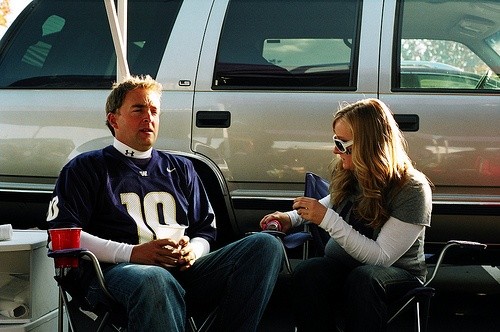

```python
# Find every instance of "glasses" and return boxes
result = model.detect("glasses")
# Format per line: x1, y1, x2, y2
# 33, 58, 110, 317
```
332, 134, 353, 153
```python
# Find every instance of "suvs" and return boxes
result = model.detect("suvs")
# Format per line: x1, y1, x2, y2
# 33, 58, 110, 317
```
1, 0, 500, 255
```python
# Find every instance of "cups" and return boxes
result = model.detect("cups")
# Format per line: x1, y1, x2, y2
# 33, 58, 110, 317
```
155, 225, 188, 267
49, 228, 83, 268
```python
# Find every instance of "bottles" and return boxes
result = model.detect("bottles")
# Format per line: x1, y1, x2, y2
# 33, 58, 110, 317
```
262, 220, 281, 231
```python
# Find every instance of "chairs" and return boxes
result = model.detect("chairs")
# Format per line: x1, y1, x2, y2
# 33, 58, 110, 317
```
46, 248, 223, 332
261, 171, 489, 332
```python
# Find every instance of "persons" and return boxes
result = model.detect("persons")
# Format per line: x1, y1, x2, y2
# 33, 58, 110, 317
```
259, 97, 432, 332
46, 74, 284, 332
